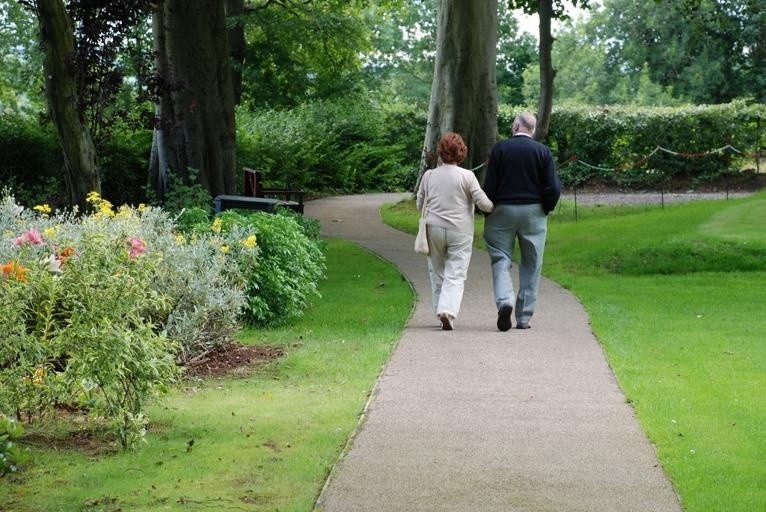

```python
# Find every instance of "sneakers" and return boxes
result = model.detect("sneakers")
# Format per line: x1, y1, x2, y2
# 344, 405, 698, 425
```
516, 321, 531, 329
439, 312, 454, 330
497, 303, 513, 331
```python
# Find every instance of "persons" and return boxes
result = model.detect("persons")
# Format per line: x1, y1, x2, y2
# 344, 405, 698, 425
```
415, 132, 494, 330
483, 111, 560, 331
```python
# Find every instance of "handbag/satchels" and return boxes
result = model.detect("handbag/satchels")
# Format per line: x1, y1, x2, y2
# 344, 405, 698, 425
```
414, 218, 429, 255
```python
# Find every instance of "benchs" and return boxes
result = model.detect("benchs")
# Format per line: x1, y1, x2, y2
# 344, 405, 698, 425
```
243, 167, 305, 215
